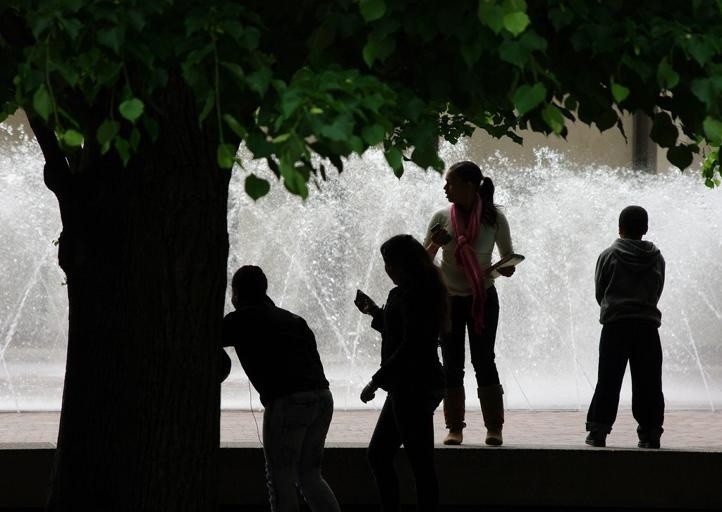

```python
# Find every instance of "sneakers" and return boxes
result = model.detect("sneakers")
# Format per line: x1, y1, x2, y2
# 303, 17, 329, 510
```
637, 437, 662, 451
584, 429, 608, 449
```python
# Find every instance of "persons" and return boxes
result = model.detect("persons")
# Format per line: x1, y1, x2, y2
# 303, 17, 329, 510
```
221, 263, 343, 512
420, 160, 516, 446
354, 235, 453, 510
585, 205, 666, 450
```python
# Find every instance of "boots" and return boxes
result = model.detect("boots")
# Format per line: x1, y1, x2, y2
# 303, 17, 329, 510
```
443, 385, 467, 445
478, 383, 505, 446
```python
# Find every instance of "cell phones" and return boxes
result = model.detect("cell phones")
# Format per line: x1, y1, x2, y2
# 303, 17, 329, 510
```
430, 222, 453, 246
